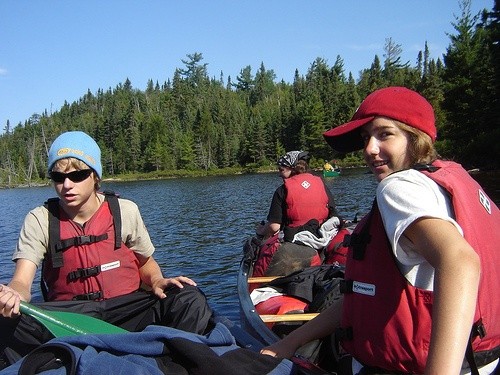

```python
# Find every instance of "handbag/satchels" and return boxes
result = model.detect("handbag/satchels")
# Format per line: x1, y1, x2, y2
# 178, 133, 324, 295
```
253, 228, 354, 315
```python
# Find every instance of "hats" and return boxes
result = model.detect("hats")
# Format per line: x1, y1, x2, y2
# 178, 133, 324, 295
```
322, 86, 437, 153
47, 131, 102, 179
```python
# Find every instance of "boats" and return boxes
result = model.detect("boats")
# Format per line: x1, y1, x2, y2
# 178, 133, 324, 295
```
236, 237, 342, 375
0, 300, 311, 375
322, 169, 341, 177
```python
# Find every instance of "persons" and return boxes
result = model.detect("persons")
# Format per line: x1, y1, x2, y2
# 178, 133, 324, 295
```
322, 160, 340, 172
260, 86, 500, 375
255, 151, 338, 243
0, 131, 214, 365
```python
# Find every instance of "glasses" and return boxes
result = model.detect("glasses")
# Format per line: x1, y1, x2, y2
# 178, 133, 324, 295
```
49, 168, 94, 183
